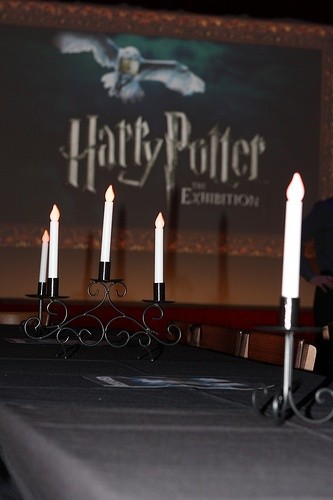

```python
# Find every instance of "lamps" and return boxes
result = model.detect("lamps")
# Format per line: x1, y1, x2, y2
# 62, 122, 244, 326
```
21, 183, 183, 349
250, 172, 332, 425
